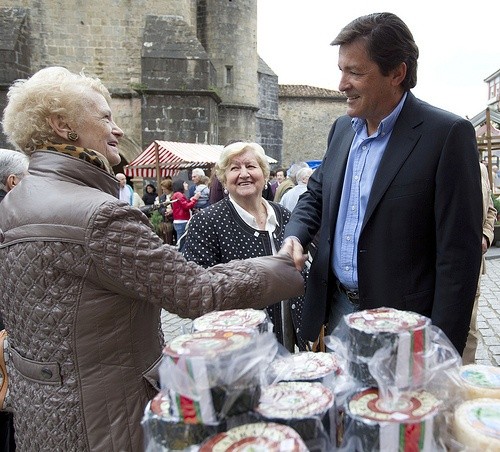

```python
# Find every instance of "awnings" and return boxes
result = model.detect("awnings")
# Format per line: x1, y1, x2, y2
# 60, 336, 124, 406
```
123, 140, 280, 202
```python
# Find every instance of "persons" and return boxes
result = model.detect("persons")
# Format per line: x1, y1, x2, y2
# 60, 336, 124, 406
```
463, 161, 498, 364
276, 11, 484, 361
179, 141, 309, 353
0, 148, 29, 203
115, 162, 319, 248
0, 67, 308, 452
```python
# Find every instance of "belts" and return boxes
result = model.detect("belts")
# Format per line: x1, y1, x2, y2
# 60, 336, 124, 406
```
335, 278, 359, 305
